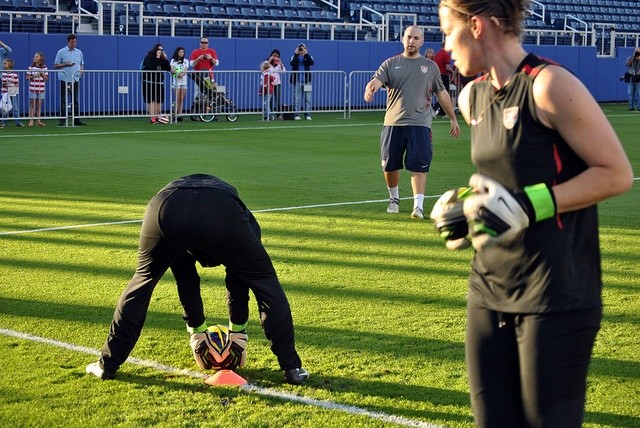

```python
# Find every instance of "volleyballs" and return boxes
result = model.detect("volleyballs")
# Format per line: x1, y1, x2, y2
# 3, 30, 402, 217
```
204, 324, 240, 372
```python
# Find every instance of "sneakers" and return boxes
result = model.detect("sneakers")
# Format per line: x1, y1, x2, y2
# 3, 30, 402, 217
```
411, 208, 424, 219
28, 123, 34, 126
75, 121, 86, 126
16, 124, 25, 127
150, 118, 157, 124
295, 116, 301, 120
306, 116, 312, 120
58, 123, 65, 126
86, 361, 115, 379
0, 124, 6, 128
37, 123, 45, 126
172, 117, 183, 121
284, 368, 309, 385
387, 198, 399, 213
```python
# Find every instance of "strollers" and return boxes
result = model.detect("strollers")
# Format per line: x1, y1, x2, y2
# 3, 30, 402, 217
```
188, 69, 238, 122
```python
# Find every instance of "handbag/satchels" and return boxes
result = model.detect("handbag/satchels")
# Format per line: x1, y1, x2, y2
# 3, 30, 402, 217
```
283, 113, 294, 120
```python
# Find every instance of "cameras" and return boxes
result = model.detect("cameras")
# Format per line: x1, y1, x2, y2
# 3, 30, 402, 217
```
635, 51, 640, 58
298, 47, 303, 51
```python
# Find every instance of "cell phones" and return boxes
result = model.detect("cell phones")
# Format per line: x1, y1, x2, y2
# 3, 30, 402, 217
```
71, 63, 75, 65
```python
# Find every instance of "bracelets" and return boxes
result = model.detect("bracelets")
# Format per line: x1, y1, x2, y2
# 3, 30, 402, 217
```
1, 44, 5, 47
452, 71, 455, 74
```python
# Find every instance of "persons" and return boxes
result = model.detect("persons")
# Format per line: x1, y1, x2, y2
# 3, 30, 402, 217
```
87, 173, 310, 384
625, 48, 640, 111
190, 37, 219, 121
259, 49, 286, 121
434, 42, 455, 118
26, 52, 48, 127
54, 35, 87, 126
364, 25, 460, 220
290, 43, 314, 121
2, 59, 25, 128
429, 0, 634, 428
259, 63, 276, 120
169, 47, 189, 121
423, 48, 439, 119
0, 39, 12, 53
142, 44, 171, 124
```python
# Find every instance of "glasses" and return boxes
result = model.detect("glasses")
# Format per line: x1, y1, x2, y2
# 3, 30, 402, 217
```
157, 49, 163, 51
201, 42, 208, 44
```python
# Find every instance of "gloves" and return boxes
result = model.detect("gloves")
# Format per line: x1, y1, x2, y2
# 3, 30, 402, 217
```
221, 321, 248, 369
430, 187, 471, 251
463, 174, 556, 252
187, 320, 220, 370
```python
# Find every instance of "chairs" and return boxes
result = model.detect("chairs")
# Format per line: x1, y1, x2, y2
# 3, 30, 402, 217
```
1, 0, 80, 34
347, 0, 589, 45
85, 1, 372, 41
